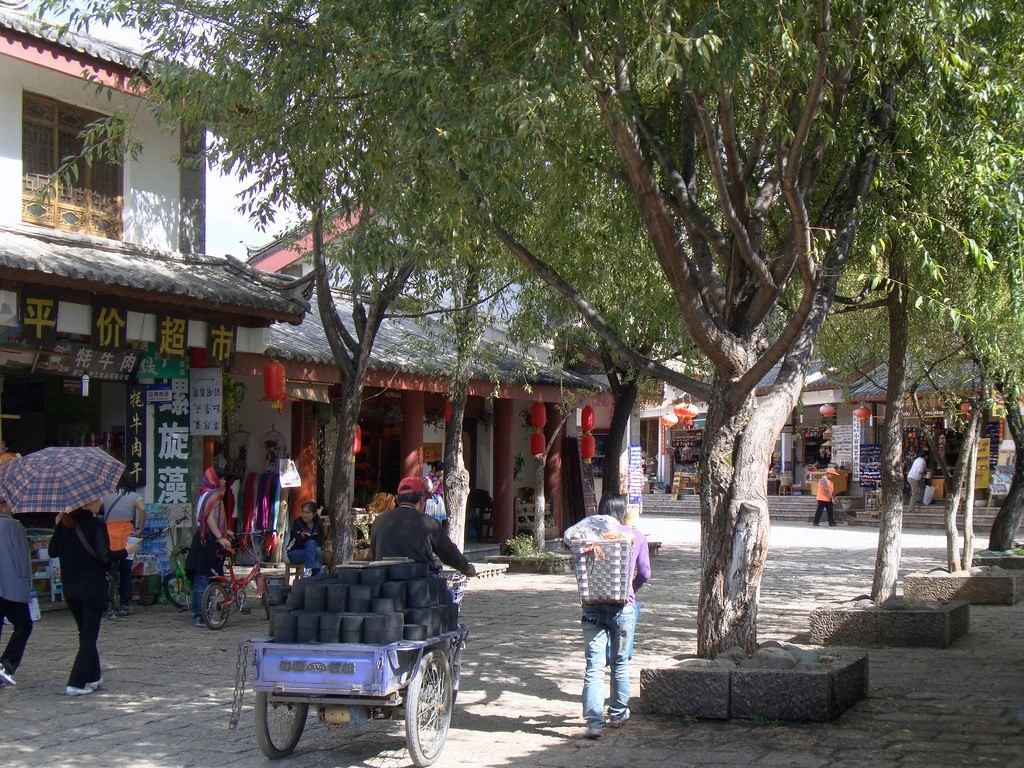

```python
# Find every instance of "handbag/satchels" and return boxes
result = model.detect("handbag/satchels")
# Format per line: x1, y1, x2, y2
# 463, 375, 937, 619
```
923, 486, 935, 505
106, 574, 114, 603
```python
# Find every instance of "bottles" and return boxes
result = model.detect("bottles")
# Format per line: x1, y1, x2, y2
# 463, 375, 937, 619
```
138, 517, 170, 573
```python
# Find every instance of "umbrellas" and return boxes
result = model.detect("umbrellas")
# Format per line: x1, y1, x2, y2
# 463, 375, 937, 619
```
1, 446, 127, 513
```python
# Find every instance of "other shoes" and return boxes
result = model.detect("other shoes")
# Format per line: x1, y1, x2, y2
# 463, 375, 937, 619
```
609, 709, 632, 728
302, 567, 314, 578
813, 522, 820, 527
118, 606, 134, 616
829, 522, 837, 526
65, 685, 94, 697
194, 618, 219, 628
0, 661, 17, 686
586, 726, 603, 737
86, 678, 104, 690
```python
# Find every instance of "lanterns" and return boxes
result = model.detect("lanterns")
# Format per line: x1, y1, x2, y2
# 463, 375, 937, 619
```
262, 359, 287, 403
529, 401, 548, 459
350, 424, 362, 459
581, 405, 597, 461
660, 401, 699, 428
444, 397, 453, 423
961, 399, 981, 419
852, 405, 873, 420
819, 403, 835, 416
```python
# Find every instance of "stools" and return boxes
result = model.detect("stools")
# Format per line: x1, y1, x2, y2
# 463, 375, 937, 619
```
284, 563, 306, 586
778, 485, 791, 496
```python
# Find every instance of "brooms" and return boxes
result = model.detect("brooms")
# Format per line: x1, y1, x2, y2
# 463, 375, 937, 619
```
832, 497, 855, 525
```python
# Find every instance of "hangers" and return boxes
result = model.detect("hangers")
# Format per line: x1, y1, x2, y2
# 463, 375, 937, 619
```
264, 424, 280, 435
232, 424, 249, 435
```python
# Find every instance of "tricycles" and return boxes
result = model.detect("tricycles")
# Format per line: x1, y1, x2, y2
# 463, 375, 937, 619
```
249, 569, 468, 768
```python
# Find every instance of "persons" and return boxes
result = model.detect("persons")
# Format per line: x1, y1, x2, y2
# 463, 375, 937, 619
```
581, 492, 652, 736
422, 460, 450, 526
812, 467, 839, 527
99, 470, 147, 617
288, 501, 328, 578
0, 495, 34, 686
184, 466, 235, 627
370, 477, 477, 579
47, 495, 143, 695
906, 450, 930, 513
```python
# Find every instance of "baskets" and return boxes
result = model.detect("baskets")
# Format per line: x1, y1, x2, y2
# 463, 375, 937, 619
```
436, 570, 465, 604
569, 539, 634, 604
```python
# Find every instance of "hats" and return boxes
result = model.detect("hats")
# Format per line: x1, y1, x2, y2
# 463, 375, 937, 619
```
398, 479, 427, 495
827, 467, 840, 477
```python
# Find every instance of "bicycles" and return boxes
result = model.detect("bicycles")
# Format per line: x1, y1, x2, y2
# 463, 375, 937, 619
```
199, 529, 277, 630
146, 515, 194, 610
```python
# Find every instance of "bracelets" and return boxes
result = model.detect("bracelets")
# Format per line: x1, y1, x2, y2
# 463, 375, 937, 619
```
217, 535, 224, 540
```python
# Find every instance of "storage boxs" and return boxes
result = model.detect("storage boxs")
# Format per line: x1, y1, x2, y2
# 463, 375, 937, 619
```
131, 573, 161, 596
136, 501, 192, 527
